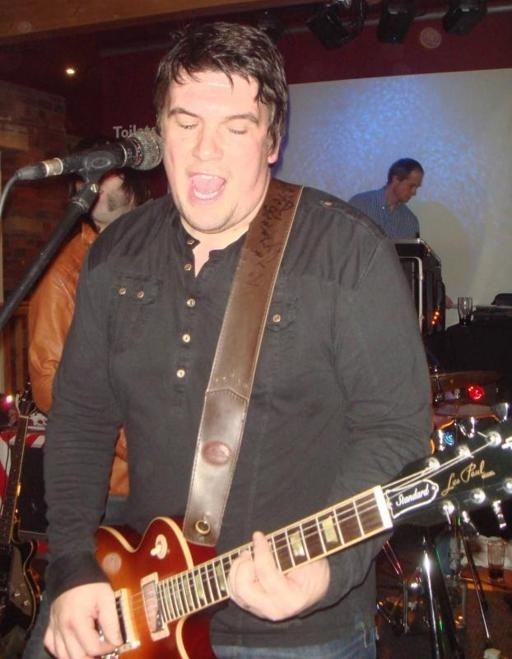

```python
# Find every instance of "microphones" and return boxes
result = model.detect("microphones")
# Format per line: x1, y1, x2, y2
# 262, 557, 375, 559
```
18, 127, 163, 182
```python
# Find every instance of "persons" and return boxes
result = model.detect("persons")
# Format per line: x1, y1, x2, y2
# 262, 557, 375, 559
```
345, 158, 425, 242
28, 131, 153, 525
43, 23, 431, 659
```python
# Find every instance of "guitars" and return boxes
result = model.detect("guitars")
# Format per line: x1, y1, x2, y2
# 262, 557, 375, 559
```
0, 380, 39, 636
94, 403, 511, 659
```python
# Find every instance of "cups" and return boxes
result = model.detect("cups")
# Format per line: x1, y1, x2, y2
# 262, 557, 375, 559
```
487, 539, 506, 583
457, 296, 473, 327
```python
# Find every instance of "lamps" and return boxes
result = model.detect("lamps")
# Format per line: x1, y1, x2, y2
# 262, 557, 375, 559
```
303, 1, 487, 50
